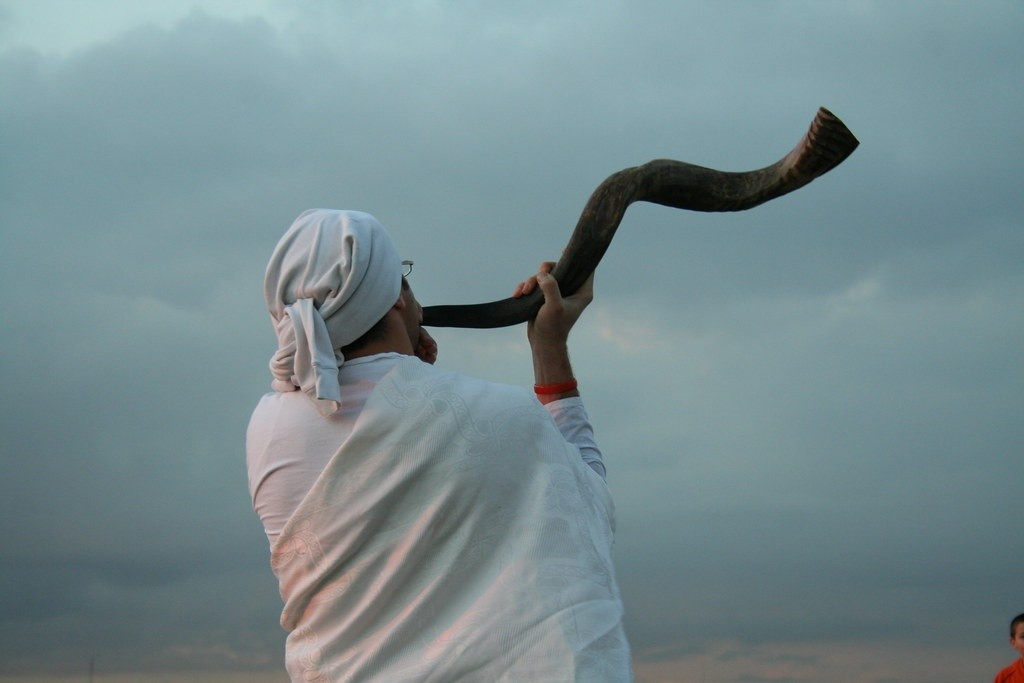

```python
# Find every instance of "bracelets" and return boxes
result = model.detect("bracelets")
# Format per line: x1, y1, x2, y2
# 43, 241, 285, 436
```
533, 377, 578, 395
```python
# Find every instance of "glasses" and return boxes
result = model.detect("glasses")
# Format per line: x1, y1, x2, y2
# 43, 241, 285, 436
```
401, 258, 414, 277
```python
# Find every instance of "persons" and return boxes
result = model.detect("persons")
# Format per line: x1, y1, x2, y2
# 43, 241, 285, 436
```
994, 613, 1024, 683
244, 209, 631, 683
